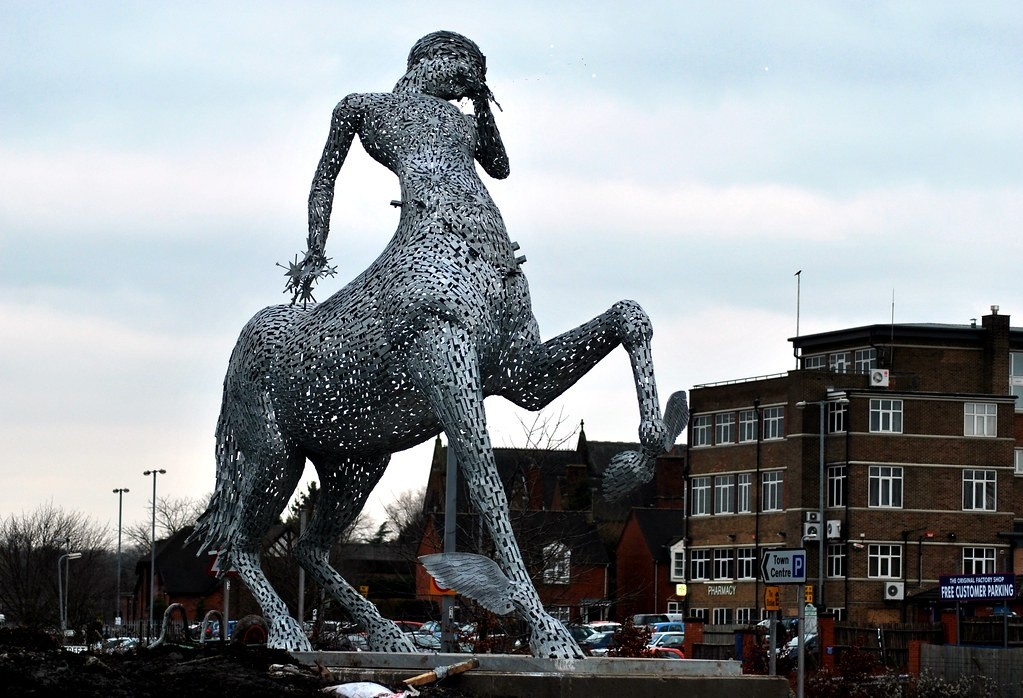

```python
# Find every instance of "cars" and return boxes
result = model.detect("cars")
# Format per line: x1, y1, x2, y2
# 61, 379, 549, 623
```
56, 630, 156, 657
187, 609, 685, 662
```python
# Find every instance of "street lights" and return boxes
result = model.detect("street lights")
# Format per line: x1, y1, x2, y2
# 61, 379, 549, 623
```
793, 398, 852, 607
111, 488, 130, 626
57, 553, 82, 628
55, 538, 68, 632
143, 469, 165, 636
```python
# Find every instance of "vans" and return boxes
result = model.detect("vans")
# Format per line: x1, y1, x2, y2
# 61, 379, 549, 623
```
765, 633, 820, 673
756, 614, 798, 644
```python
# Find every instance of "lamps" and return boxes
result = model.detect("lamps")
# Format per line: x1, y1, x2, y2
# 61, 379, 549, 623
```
948, 533, 956, 539
777, 532, 786, 537
727, 535, 736, 541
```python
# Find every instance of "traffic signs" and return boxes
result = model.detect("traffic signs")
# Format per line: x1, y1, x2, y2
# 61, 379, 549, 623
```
759, 547, 806, 584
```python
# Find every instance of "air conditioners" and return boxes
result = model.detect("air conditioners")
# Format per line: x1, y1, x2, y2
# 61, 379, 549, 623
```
803, 523, 820, 540
869, 369, 889, 387
884, 582, 904, 600
826, 520, 841, 538
806, 512, 820, 522
